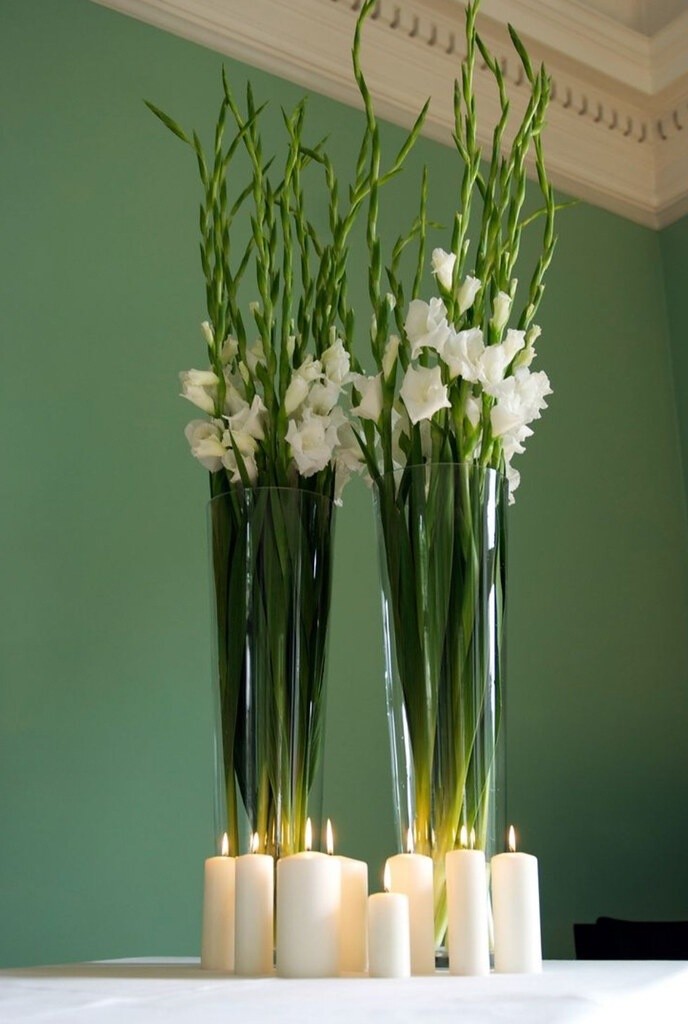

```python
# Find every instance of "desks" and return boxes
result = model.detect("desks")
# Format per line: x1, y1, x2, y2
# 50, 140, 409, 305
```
0, 956, 688, 1024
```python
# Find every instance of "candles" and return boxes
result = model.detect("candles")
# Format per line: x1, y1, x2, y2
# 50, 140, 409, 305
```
200, 816, 543, 978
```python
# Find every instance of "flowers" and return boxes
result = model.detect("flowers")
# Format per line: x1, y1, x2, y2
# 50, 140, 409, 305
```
141, 59, 433, 507
348, 1, 588, 507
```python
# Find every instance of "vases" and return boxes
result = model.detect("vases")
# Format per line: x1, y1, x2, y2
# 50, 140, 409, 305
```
205, 484, 339, 971
369, 461, 509, 969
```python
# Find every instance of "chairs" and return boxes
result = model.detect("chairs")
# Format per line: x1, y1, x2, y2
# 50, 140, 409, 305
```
573, 915, 688, 960
